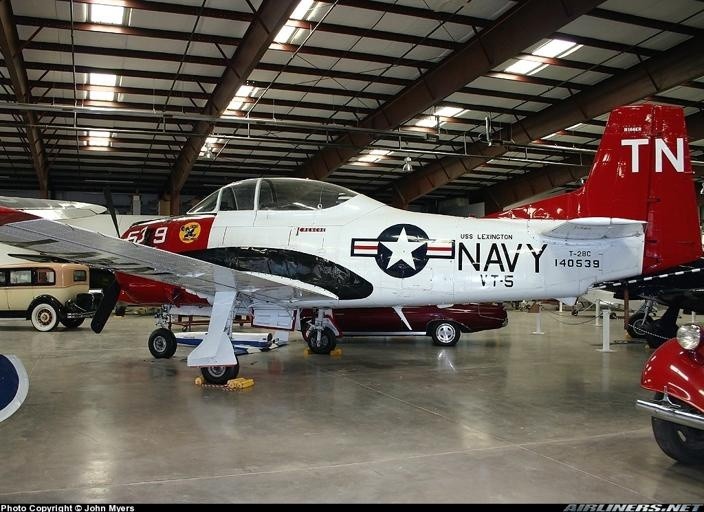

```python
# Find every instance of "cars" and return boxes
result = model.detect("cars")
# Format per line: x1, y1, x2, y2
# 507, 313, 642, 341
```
295, 300, 510, 348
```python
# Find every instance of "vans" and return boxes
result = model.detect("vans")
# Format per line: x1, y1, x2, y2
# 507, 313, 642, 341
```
0, 261, 92, 333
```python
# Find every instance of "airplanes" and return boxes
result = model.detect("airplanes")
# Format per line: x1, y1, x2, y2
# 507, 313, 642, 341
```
0, 98, 704, 390
